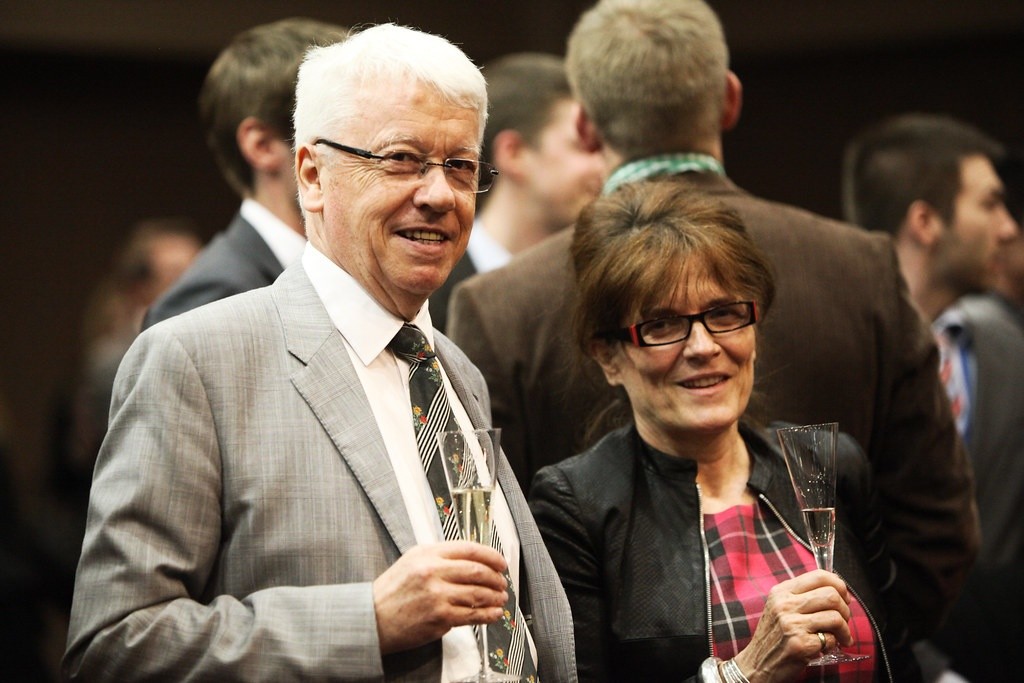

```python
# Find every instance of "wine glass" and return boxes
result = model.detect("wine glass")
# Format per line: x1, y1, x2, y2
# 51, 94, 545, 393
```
776, 420, 872, 667
435, 428, 523, 683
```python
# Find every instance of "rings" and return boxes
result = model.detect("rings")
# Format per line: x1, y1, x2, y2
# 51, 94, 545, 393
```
816, 632, 827, 648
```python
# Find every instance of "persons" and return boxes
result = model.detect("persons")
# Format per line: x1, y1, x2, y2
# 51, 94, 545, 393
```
837, 116, 1023, 682
450, 0, 970, 622
40, 0, 616, 683
524, 178, 921, 683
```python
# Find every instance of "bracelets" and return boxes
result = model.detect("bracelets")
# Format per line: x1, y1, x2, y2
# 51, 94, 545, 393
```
700, 656, 751, 683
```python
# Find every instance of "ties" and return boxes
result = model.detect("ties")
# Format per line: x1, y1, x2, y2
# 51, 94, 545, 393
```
387, 323, 536, 683
934, 333, 961, 430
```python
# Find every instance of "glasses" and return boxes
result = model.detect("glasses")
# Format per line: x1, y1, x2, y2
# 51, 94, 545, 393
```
593, 300, 758, 348
313, 137, 497, 193
